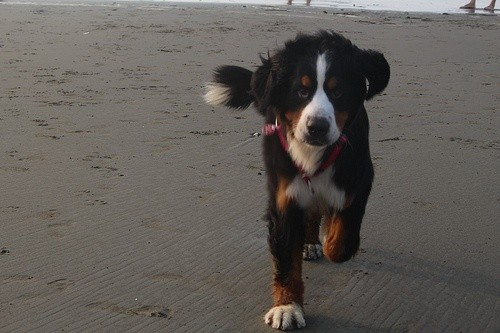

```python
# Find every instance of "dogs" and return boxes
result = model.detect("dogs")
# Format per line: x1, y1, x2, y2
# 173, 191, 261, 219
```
201, 28, 391, 331
